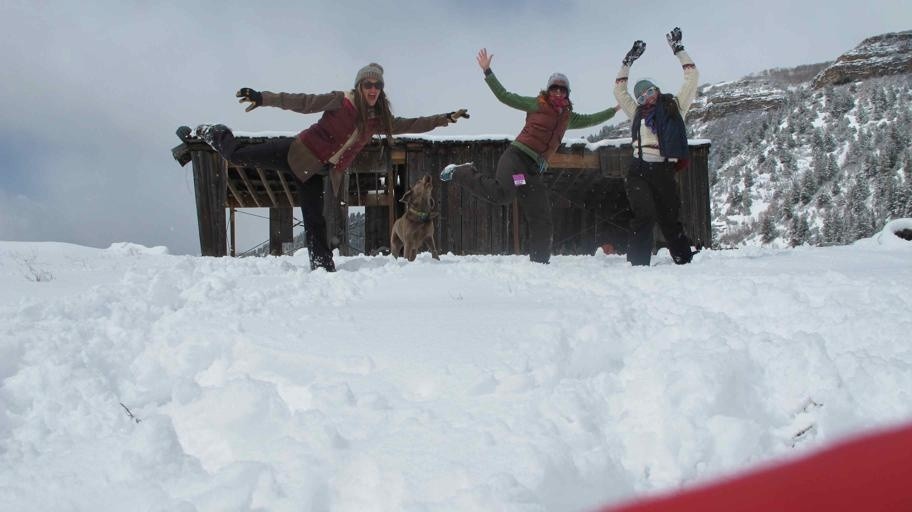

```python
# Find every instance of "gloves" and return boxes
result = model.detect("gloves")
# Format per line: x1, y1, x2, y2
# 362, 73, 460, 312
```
666, 27, 684, 56
236, 88, 263, 112
446, 110, 470, 123
622, 40, 646, 67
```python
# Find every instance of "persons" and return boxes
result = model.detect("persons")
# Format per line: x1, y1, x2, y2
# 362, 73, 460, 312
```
195, 61, 470, 273
439, 46, 622, 262
614, 26, 699, 269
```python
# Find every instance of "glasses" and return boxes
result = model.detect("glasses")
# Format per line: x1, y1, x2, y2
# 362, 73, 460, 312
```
361, 81, 383, 89
636, 87, 655, 105
549, 84, 567, 93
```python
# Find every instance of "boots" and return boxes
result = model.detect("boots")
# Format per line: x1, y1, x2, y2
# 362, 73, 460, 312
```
309, 248, 335, 272
188, 125, 232, 151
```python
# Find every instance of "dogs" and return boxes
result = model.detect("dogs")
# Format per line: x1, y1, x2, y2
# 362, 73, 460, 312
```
388, 174, 440, 261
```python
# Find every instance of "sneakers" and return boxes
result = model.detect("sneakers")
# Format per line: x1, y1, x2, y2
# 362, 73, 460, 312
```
439, 161, 478, 182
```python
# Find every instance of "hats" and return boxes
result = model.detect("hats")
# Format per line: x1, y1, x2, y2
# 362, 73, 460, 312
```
355, 63, 384, 90
634, 80, 653, 99
547, 73, 571, 96
